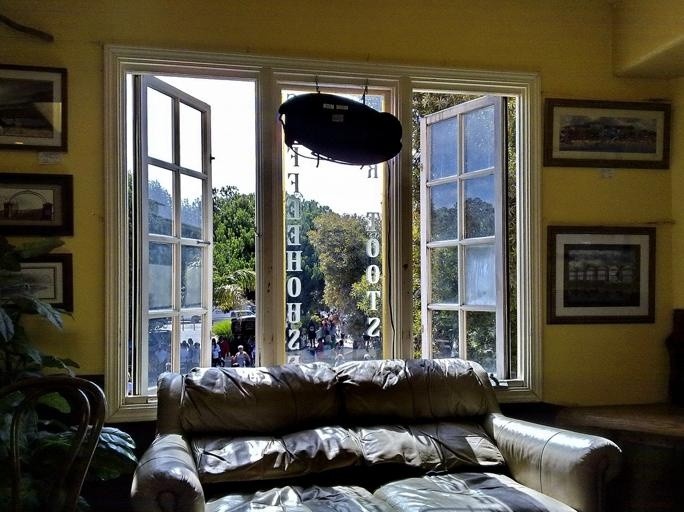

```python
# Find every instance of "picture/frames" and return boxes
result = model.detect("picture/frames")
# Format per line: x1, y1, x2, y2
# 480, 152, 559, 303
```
545, 223, 656, 326
0, 172, 75, 239
542, 96, 672, 170
0, 251, 75, 315
0, 62, 69, 154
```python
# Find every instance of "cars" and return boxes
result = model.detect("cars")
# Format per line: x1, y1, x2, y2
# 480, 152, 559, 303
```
180, 305, 256, 324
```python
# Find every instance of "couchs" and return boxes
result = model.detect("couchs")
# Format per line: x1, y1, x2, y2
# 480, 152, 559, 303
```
129, 357, 624, 512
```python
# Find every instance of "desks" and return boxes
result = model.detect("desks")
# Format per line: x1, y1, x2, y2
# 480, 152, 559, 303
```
556, 404, 684, 512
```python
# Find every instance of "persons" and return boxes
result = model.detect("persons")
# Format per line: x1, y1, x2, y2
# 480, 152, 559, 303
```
440, 336, 452, 358
285, 311, 380, 357
482, 350, 496, 372
156, 338, 255, 374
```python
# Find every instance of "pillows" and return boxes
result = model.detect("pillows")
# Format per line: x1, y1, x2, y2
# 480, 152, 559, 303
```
189, 424, 366, 486
354, 418, 506, 475
